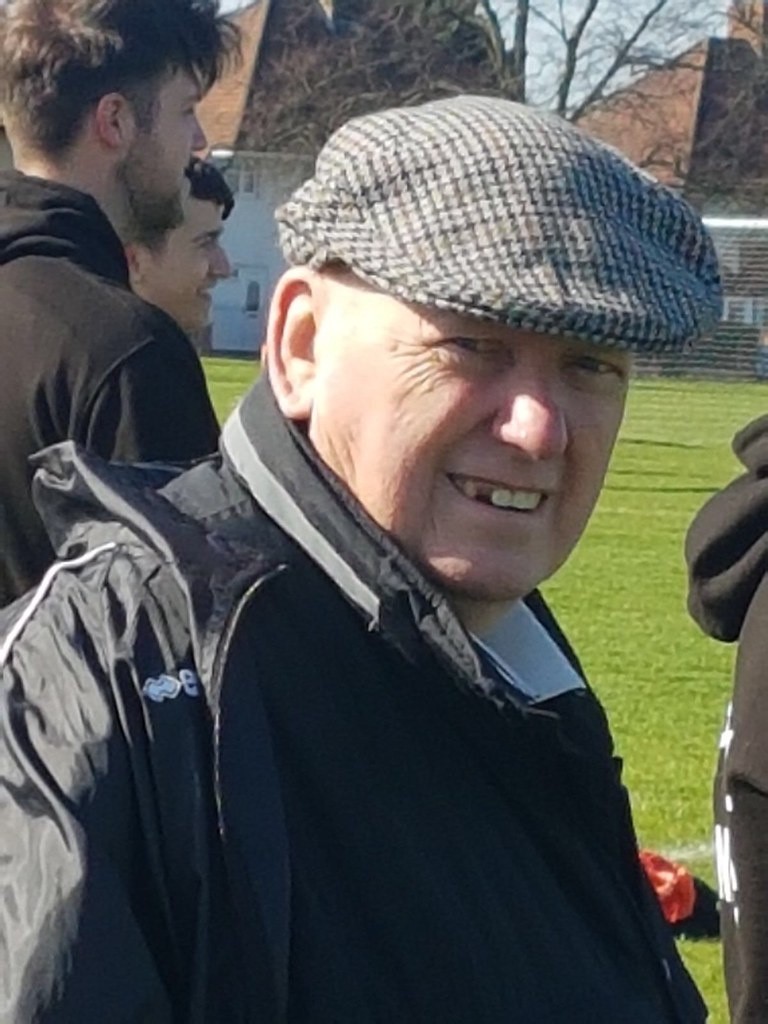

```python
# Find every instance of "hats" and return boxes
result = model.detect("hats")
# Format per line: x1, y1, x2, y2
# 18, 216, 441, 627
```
274, 94, 723, 351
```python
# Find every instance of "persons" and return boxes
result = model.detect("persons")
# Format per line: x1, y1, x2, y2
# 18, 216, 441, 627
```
0, 93, 728, 1023
681, 410, 767, 1023
0, 1, 237, 610
